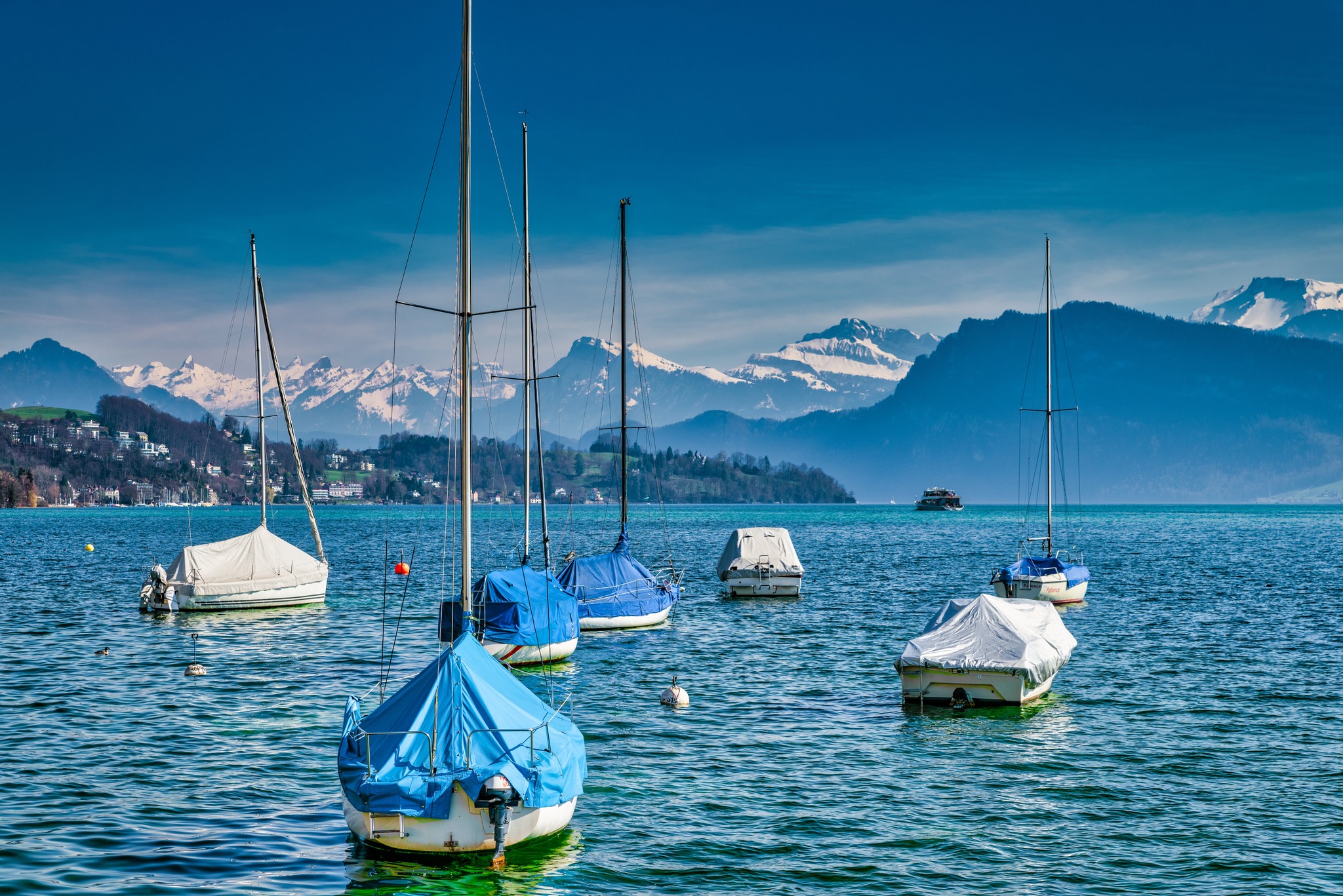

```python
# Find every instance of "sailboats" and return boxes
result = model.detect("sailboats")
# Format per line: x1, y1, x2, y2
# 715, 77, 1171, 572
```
434, 108, 585, 667
987, 231, 1090, 606
554, 194, 686, 632
330, 0, 589, 865
137, 228, 330, 613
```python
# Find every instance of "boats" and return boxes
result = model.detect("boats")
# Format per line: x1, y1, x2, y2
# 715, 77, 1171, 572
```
715, 527, 805, 598
891, 592, 1079, 716
913, 484, 965, 511
